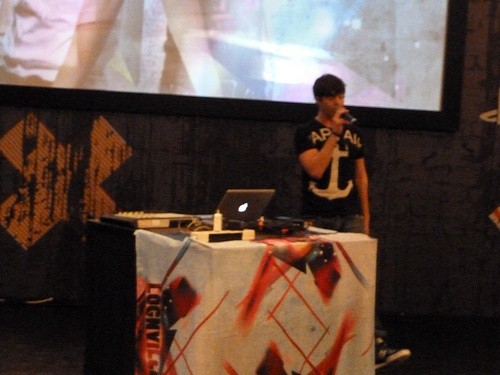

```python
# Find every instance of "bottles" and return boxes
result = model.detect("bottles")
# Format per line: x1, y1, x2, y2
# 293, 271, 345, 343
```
213, 209, 222, 231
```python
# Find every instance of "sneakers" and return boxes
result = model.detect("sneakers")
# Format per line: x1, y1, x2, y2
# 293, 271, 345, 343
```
375, 338, 412, 371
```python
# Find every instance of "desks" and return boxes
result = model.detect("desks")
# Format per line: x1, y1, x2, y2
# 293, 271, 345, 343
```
86, 219, 379, 375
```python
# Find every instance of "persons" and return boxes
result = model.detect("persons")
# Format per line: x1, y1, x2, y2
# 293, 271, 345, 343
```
292, 74, 411, 371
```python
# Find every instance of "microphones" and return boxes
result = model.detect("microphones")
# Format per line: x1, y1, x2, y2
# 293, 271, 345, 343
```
342, 113, 357, 123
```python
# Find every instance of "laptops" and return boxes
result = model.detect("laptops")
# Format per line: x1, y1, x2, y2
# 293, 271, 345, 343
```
196, 189, 276, 222
